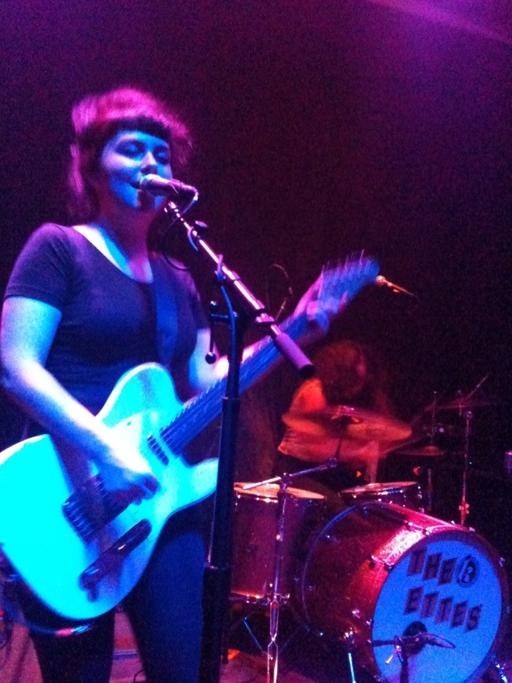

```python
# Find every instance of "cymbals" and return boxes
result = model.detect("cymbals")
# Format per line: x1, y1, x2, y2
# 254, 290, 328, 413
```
445, 398, 490, 408
394, 446, 446, 457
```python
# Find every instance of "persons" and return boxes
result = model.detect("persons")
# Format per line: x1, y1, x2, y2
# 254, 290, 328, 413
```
0, 86, 353, 683
276, 336, 390, 480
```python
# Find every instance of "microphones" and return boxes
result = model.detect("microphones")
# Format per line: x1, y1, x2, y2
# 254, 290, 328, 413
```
140, 173, 199, 198
376, 275, 409, 296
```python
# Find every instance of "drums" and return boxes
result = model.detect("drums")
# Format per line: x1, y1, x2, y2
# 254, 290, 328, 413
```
294, 497, 509, 683
227, 481, 326, 610
341, 481, 422, 509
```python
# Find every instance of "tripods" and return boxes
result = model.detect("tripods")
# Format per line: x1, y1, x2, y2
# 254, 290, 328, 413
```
235, 455, 340, 682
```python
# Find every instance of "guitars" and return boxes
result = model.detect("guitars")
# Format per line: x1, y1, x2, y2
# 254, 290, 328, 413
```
0, 249, 379, 620
